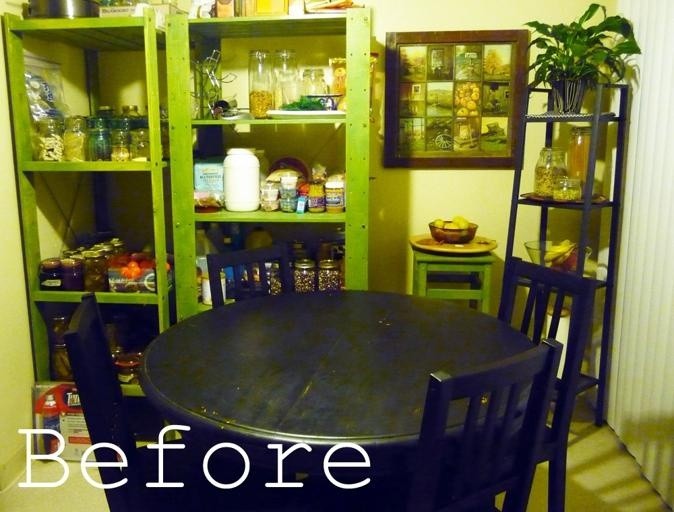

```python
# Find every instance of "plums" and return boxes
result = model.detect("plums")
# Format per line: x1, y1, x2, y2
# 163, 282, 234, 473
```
432, 216, 470, 237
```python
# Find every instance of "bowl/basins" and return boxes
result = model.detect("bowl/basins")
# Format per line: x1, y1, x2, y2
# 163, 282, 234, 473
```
523, 240, 593, 275
27, 0, 99, 18
429, 221, 479, 244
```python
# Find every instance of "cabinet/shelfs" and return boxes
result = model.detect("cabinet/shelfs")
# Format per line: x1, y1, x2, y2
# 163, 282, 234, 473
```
1, 6, 176, 462
164, 7, 372, 324
503, 84, 632, 427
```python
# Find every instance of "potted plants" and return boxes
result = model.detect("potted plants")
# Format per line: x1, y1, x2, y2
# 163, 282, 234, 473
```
523, 2, 641, 116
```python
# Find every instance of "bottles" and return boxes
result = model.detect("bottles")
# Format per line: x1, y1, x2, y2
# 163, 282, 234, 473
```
566, 126, 605, 199
196, 224, 345, 303
247, 47, 344, 117
35, 105, 169, 166
41, 394, 61, 454
534, 149, 566, 198
214, 98, 237, 115
222, 146, 344, 214
552, 179, 583, 203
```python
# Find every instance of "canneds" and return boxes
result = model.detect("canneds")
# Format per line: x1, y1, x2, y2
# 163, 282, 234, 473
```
39, 105, 344, 386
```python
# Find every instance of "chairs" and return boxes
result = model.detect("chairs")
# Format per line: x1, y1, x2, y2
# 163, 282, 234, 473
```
394, 336, 563, 511
207, 237, 294, 307
62, 293, 261, 512
492, 254, 597, 511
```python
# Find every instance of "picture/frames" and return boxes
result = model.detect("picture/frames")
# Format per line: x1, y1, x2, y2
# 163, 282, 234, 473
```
383, 29, 528, 169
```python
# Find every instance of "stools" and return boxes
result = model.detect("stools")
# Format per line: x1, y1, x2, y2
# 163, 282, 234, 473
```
412, 250, 497, 316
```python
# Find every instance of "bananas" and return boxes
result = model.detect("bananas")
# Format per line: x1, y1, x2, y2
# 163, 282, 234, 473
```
543, 239, 577, 265
